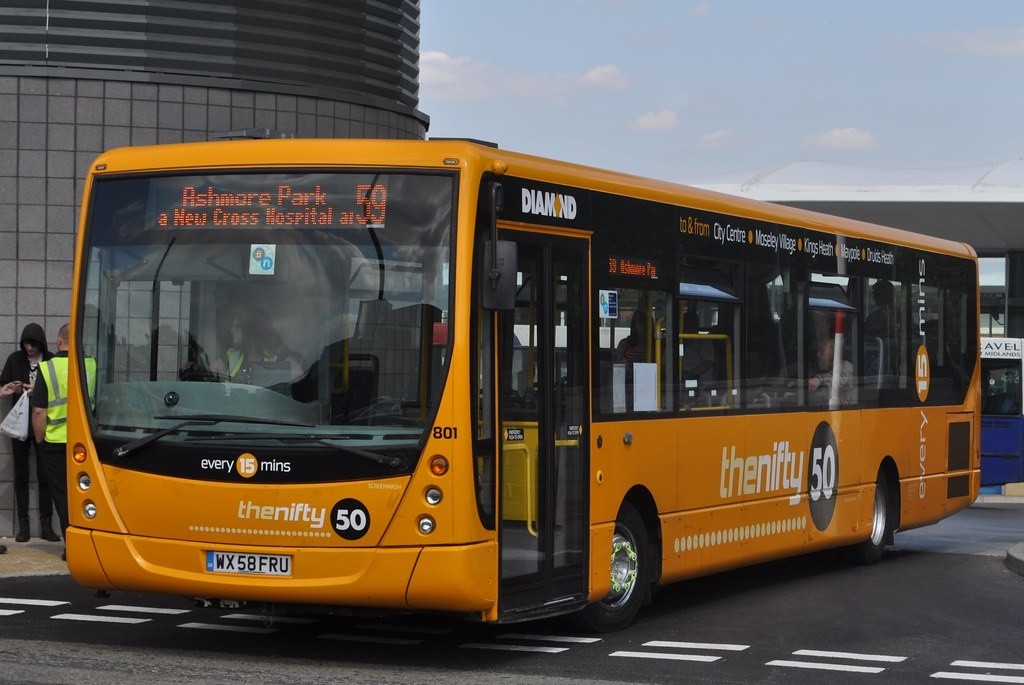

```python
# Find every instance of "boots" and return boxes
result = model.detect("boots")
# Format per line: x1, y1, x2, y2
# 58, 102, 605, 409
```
16, 516, 30, 542
40, 517, 60, 541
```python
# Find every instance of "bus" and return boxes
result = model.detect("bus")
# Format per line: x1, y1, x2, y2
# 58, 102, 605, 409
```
60, 139, 984, 638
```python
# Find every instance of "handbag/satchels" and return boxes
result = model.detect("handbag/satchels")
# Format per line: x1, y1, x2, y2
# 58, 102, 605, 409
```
0, 391, 30, 441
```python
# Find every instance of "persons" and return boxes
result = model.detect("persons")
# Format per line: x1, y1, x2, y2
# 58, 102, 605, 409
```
30, 324, 97, 560
864, 279, 915, 337
180, 307, 307, 399
615, 308, 713, 390
808, 339, 853, 405
0, 322, 62, 542
0, 382, 18, 555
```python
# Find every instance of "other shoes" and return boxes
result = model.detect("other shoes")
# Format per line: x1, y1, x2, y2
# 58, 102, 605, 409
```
0, 545, 8, 554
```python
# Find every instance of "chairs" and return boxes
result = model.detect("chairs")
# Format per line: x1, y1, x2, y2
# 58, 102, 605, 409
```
329, 353, 379, 425
